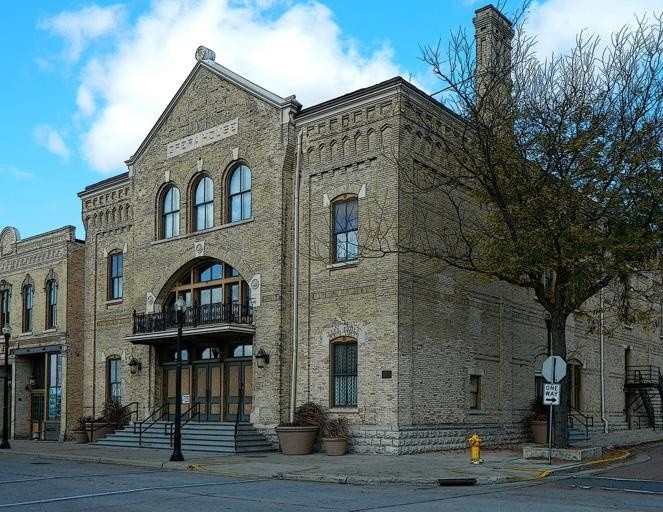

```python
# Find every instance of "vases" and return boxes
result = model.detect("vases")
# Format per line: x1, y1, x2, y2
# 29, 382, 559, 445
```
530, 421, 547, 443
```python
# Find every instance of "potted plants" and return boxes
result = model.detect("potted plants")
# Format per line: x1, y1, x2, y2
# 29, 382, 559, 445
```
322, 419, 348, 456
72, 416, 89, 443
86, 400, 131, 442
276, 401, 329, 455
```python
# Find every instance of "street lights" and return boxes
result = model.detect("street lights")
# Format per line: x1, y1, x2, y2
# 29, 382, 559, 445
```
0, 323, 12, 449
541, 309, 553, 446
170, 296, 187, 462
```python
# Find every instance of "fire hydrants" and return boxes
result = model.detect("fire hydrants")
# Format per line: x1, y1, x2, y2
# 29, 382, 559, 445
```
467, 432, 482, 463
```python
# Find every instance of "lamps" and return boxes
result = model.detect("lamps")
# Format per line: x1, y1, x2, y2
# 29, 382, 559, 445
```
255, 348, 270, 368
127, 358, 141, 374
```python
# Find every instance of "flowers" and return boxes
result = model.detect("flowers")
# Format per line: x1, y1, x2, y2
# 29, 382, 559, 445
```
530, 396, 547, 421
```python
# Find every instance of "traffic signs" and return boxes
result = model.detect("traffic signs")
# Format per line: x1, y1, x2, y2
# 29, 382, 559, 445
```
543, 384, 560, 405
